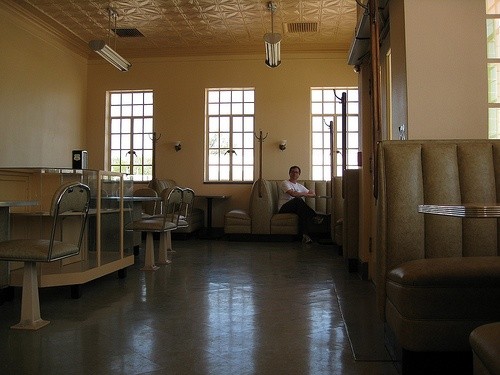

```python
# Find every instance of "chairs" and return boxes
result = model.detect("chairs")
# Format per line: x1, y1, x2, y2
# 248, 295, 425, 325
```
0, 183, 91, 330
124, 186, 195, 272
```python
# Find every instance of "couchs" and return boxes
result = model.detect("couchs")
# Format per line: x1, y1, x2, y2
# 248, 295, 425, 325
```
148, 180, 204, 233
376, 139, 500, 353
224, 178, 332, 235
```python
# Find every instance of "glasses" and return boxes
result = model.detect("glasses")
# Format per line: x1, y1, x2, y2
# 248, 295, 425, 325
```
290, 171, 300, 174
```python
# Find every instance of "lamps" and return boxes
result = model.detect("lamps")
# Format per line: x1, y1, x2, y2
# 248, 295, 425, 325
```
263, 1, 281, 67
89, 7, 131, 73
279, 140, 287, 151
174, 141, 181, 151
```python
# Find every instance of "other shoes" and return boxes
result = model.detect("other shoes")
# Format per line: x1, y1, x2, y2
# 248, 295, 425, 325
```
314, 215, 323, 224
303, 237, 312, 244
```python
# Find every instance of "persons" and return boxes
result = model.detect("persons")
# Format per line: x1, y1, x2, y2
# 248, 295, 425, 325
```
278, 166, 324, 244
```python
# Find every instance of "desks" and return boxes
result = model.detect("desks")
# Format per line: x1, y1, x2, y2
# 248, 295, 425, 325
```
417, 203, 500, 218
91, 194, 161, 202
194, 194, 230, 240
10, 208, 133, 218
